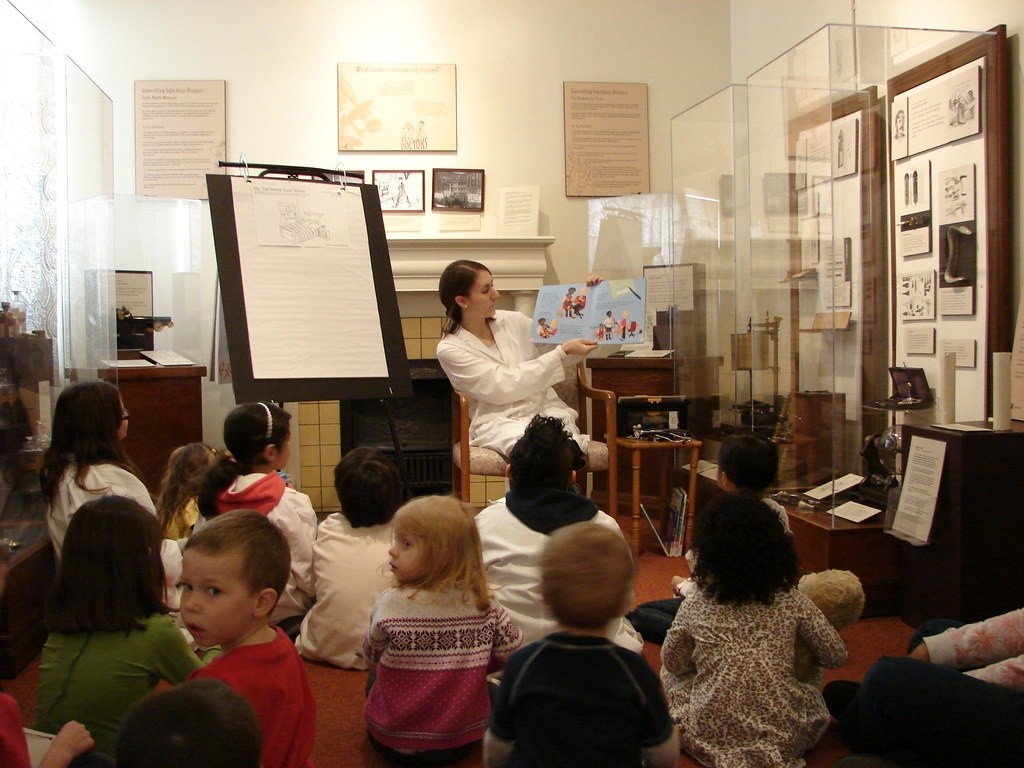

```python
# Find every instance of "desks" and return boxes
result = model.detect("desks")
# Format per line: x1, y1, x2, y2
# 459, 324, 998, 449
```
65, 351, 206, 493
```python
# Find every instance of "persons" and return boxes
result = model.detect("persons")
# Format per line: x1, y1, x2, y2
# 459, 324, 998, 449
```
0, 380, 317, 767
359, 494, 523, 768
659, 494, 847, 768
433, 260, 622, 462
481, 522, 681, 768
822, 601, 1024, 768
473, 415, 647, 655
292, 446, 407, 671
632, 434, 791, 644
176, 507, 318, 768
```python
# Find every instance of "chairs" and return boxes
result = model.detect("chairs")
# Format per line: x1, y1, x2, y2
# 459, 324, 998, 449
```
452, 362, 617, 521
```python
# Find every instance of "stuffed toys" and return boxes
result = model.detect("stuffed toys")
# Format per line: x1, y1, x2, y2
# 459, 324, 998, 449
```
793, 568, 864, 680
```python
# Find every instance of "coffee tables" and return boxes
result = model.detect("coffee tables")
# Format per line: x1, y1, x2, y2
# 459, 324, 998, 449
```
603, 432, 701, 557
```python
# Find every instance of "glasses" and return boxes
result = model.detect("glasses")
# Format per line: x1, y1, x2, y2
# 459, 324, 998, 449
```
121, 409, 132, 421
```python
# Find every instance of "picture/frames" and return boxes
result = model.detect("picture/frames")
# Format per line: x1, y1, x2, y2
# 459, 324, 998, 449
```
368, 168, 484, 212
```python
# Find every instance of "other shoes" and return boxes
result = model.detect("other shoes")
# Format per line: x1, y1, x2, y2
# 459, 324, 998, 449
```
822, 679, 861, 716
